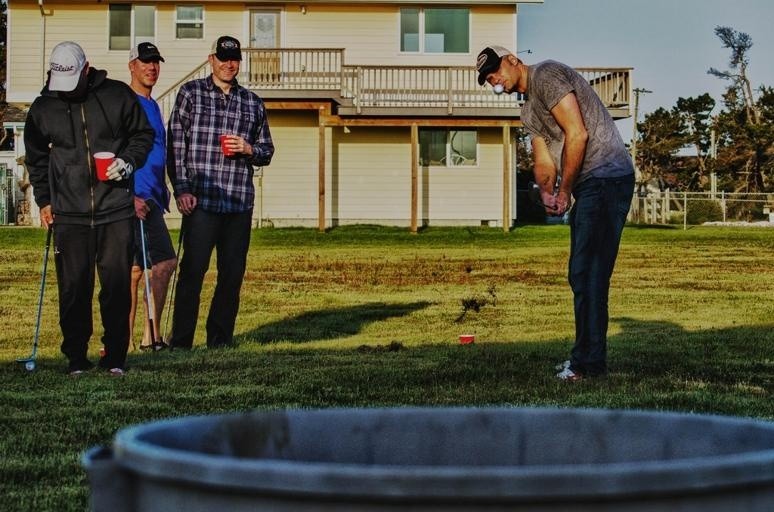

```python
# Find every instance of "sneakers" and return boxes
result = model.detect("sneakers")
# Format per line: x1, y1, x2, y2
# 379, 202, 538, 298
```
138, 337, 167, 351
68, 368, 90, 377
553, 360, 586, 382
108, 368, 124, 377
97, 346, 105, 357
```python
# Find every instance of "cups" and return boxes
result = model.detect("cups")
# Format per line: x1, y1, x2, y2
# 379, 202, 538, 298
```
459, 335, 475, 345
219, 134, 235, 156
93, 150, 115, 181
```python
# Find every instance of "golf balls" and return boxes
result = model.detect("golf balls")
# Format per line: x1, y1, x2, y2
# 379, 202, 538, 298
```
26, 362, 35, 371
494, 84, 504, 94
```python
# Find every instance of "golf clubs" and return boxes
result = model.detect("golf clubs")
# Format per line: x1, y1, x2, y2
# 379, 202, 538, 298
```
14, 214, 56, 364
162, 212, 182, 343
140, 216, 157, 370
526, 181, 557, 212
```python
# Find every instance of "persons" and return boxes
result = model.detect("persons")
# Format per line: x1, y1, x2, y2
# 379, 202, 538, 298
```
476, 45, 635, 385
165, 37, 275, 351
101, 42, 179, 357
23, 40, 155, 375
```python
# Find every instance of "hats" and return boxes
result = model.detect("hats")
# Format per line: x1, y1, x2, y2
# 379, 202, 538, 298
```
475, 45, 513, 86
208, 36, 242, 62
47, 41, 86, 93
127, 42, 165, 64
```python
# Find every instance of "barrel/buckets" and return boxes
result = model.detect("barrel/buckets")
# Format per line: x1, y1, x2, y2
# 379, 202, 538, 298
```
71, 408, 774, 509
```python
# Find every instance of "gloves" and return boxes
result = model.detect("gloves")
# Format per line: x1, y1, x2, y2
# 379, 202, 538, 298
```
105, 158, 134, 182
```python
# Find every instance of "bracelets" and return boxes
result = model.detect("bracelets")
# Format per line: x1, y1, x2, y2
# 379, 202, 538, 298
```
559, 189, 572, 195
